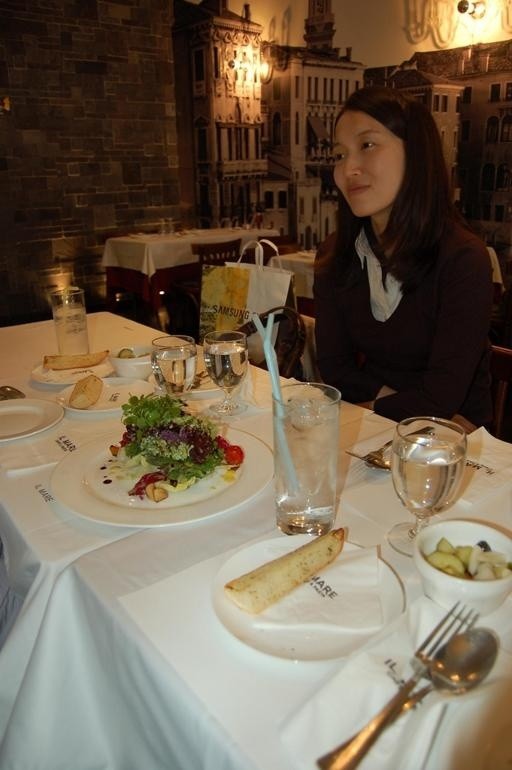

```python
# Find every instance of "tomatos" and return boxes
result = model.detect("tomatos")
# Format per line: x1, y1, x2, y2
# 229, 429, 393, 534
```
214, 435, 244, 467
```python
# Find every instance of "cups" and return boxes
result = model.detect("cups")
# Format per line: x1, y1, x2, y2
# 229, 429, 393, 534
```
152, 335, 198, 399
242, 223, 252, 233
269, 382, 342, 537
50, 288, 89, 356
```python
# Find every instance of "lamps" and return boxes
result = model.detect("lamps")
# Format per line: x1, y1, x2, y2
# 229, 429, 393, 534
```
457, 0, 487, 20
228, 4, 273, 85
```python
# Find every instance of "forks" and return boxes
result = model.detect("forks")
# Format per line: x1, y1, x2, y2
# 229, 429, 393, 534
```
324, 598, 483, 770
362, 425, 435, 463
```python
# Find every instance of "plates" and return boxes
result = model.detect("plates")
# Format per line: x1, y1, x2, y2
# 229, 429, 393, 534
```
32, 358, 115, 385
211, 534, 405, 663
50, 427, 277, 528
148, 356, 223, 393
0, 400, 65, 442
56, 376, 154, 413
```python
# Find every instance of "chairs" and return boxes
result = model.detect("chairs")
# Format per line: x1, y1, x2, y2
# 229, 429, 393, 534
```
247, 235, 293, 263
170, 238, 242, 334
217, 308, 306, 378
112, 286, 170, 334
489, 345, 512, 439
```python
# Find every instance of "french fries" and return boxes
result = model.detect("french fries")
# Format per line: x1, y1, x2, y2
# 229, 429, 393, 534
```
43, 348, 110, 372
223, 528, 345, 614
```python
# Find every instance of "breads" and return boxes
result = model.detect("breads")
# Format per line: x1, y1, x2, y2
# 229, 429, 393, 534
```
69, 374, 103, 410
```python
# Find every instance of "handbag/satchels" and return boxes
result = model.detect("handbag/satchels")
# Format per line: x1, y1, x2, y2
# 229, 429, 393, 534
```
208, 306, 307, 383
194, 237, 300, 364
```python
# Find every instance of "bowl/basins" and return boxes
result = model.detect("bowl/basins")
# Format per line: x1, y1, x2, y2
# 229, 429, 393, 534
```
110, 344, 154, 380
412, 518, 512, 619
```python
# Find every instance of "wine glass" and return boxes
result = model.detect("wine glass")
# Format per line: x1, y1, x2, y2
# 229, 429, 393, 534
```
204, 330, 249, 415
385, 416, 467, 556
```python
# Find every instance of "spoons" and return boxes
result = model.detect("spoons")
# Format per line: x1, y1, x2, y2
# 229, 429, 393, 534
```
318, 626, 499, 770
0, 386, 26, 399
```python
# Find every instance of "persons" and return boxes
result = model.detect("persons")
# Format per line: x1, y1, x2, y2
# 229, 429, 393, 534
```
313, 85, 494, 435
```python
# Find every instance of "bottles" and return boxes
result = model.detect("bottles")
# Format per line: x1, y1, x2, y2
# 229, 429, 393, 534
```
158, 218, 176, 240
